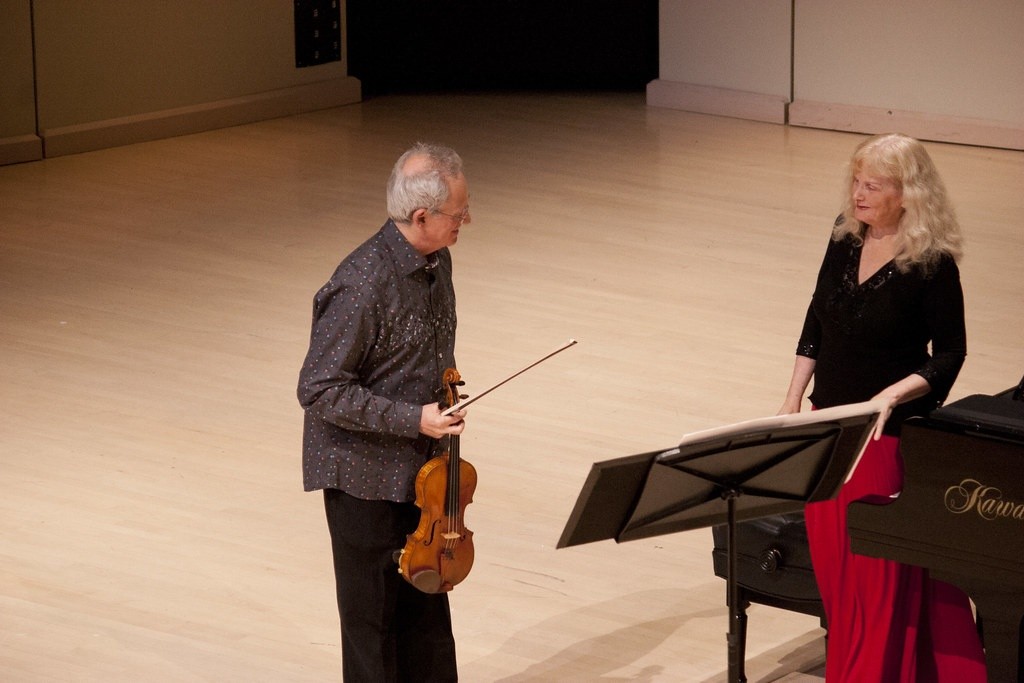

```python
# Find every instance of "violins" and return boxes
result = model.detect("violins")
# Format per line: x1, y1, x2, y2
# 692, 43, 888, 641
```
397, 365, 479, 597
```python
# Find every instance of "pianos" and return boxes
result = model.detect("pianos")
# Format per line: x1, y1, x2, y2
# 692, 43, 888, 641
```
712, 370, 1024, 683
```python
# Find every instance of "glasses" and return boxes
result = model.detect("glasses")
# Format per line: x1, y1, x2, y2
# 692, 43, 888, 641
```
411, 192, 470, 224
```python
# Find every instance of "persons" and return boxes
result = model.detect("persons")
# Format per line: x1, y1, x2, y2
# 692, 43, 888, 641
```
778, 133, 967, 683
297, 142, 473, 682
